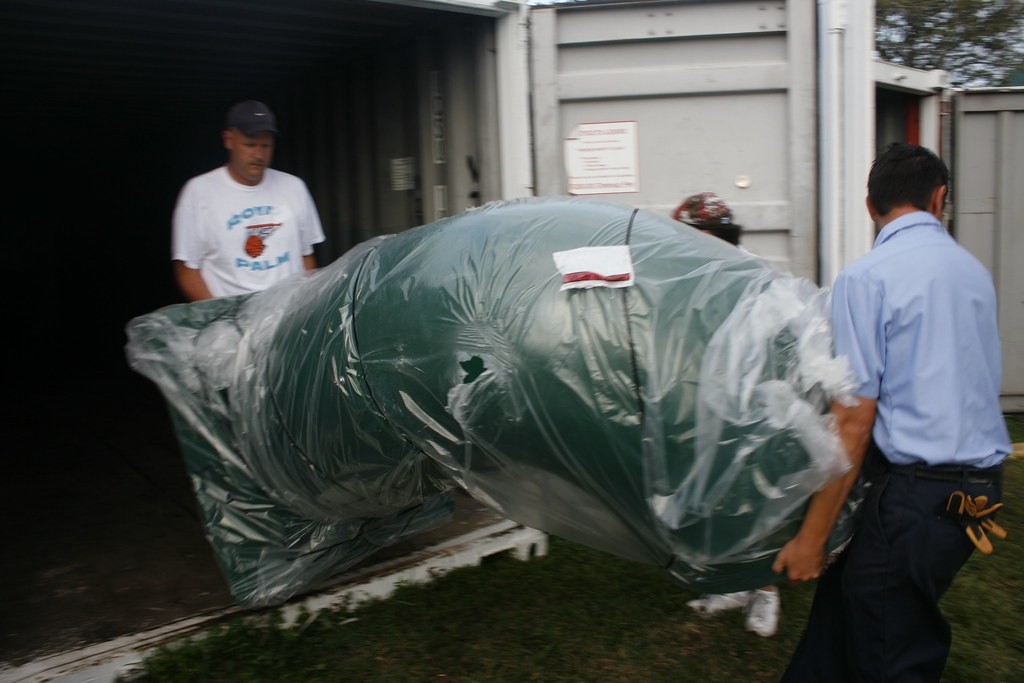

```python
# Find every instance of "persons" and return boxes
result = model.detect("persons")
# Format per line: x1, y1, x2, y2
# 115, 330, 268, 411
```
171, 99, 328, 308
670, 191, 783, 638
767, 141, 1013, 683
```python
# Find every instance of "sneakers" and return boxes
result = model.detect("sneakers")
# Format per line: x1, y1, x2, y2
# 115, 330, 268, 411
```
688, 591, 752, 617
745, 587, 782, 637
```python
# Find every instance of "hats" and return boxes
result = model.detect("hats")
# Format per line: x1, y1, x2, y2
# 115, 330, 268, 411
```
675, 193, 740, 242
226, 99, 279, 136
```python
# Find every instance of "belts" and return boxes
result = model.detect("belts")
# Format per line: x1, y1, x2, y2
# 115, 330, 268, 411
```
893, 463, 1008, 484
